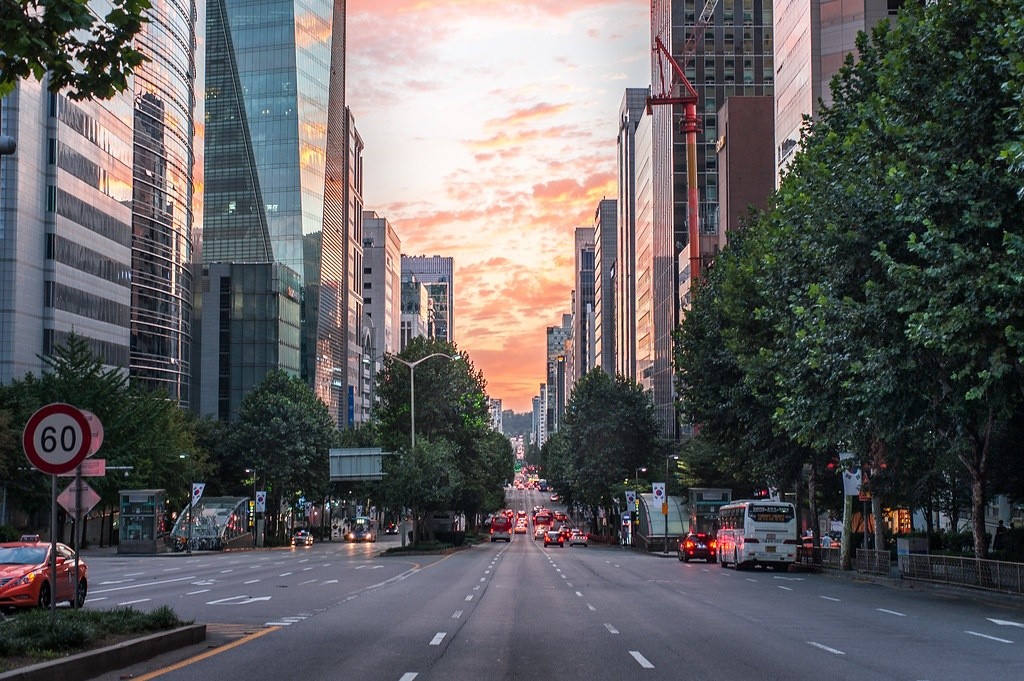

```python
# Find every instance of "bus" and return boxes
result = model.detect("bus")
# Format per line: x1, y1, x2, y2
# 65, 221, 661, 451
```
533, 512, 551, 530
348, 517, 377, 543
540, 509, 554, 528
716, 499, 797, 572
490, 517, 512, 542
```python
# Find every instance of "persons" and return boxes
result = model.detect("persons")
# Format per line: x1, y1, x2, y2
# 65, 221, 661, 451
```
388, 520, 394, 535
822, 532, 832, 548
996, 520, 1014, 535
806, 528, 812, 537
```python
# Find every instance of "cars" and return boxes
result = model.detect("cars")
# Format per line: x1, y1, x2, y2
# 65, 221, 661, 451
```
557, 514, 567, 522
802, 537, 840, 556
534, 524, 549, 540
516, 518, 528, 527
568, 530, 588, 547
0, 535, 88, 614
516, 511, 528, 521
518, 485, 524, 490
677, 531, 717, 563
544, 531, 563, 547
553, 511, 563, 518
291, 532, 313, 546
528, 484, 534, 490
385, 524, 399, 535
550, 495, 558, 501
514, 524, 526, 534
558, 526, 571, 541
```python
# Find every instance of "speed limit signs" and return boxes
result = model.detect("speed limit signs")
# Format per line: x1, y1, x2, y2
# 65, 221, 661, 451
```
22, 403, 91, 474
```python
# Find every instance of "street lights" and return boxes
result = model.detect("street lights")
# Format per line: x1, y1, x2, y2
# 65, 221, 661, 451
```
180, 453, 191, 554
363, 353, 462, 544
245, 466, 256, 548
635, 467, 647, 534
664, 454, 678, 554
